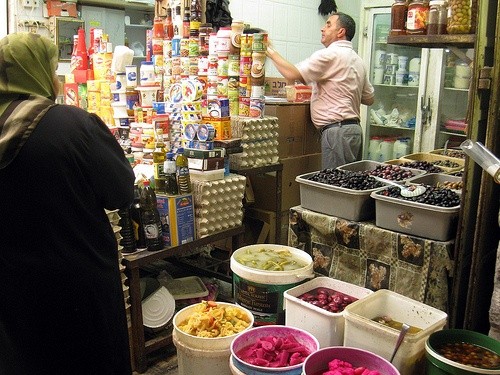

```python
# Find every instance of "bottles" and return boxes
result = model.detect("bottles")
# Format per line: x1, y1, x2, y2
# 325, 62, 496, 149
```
129, 185, 147, 249
119, 208, 137, 254
164, 153, 178, 196
175, 147, 190, 196
369, 24, 420, 164
153, 142, 167, 194
141, 181, 164, 251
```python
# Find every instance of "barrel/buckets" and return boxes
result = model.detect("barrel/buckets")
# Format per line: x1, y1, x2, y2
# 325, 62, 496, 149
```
227, 324, 320, 375
301, 345, 400, 375
419, 326, 500, 375
283, 277, 374, 349
230, 243, 314, 328
343, 288, 448, 375
171, 301, 254, 375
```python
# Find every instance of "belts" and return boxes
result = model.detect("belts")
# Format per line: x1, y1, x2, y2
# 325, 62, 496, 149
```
321, 119, 359, 132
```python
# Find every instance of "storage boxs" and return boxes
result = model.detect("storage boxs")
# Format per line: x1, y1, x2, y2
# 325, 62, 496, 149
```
285, 86, 312, 103
243, 206, 275, 245
296, 149, 466, 241
63, 56, 237, 182
265, 77, 295, 97
157, 191, 196, 247
266, 104, 321, 246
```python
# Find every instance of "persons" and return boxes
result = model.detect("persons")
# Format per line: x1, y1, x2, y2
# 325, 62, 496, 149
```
258, 9, 376, 173
0, 30, 138, 375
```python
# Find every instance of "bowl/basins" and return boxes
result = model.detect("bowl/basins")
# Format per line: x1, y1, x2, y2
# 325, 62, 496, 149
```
452, 65, 472, 89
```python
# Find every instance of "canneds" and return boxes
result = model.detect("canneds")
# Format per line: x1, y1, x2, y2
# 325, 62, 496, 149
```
184, 123, 215, 150
77, 51, 153, 128
152, 19, 268, 120
373, 49, 421, 87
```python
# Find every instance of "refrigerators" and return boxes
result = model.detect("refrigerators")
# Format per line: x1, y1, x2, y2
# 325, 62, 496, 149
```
356, 0, 474, 164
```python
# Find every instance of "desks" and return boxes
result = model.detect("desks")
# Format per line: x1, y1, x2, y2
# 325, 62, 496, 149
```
122, 224, 247, 374
160, 162, 284, 284
289, 205, 454, 325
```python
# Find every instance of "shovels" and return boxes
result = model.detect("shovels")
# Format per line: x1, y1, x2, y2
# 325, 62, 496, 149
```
370, 175, 426, 198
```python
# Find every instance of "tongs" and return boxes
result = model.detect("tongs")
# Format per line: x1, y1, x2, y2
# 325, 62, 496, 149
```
388, 324, 410, 363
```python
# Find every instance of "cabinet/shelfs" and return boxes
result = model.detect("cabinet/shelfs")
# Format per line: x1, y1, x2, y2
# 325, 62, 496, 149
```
357, 0, 474, 163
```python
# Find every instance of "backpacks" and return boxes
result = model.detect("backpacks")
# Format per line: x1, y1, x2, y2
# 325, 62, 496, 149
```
206, 0, 232, 26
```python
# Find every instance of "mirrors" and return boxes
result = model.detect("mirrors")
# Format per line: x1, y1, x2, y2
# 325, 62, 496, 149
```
56, 17, 86, 61
